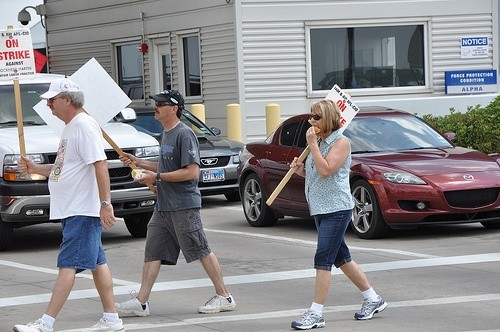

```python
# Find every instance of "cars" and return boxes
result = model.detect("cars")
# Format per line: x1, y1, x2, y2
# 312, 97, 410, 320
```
235, 103, 500, 241
110, 104, 246, 204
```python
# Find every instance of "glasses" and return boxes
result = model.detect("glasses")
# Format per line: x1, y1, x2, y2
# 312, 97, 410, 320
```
309, 114, 322, 121
46, 97, 66, 102
152, 103, 175, 108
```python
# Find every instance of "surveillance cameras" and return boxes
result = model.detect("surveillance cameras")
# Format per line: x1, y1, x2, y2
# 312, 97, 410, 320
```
18, 10, 31, 25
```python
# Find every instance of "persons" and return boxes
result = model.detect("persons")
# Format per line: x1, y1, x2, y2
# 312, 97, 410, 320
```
115, 90, 237, 315
12, 78, 124, 331
289, 100, 387, 330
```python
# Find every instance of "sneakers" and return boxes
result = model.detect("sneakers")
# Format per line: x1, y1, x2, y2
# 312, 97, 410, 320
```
90, 318, 124, 332
291, 308, 325, 330
13, 319, 53, 332
115, 290, 150, 316
198, 293, 236, 313
354, 296, 387, 320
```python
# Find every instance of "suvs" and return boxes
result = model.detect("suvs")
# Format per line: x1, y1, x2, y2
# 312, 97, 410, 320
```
0, 70, 162, 248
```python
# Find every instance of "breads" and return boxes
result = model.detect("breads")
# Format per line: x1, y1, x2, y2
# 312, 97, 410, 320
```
314, 126, 321, 134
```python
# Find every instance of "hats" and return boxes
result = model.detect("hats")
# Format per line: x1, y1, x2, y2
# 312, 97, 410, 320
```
149, 89, 185, 110
39, 78, 80, 99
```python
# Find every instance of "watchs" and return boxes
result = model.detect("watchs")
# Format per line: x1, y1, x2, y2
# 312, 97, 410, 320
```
155, 172, 162, 183
101, 201, 111, 207
